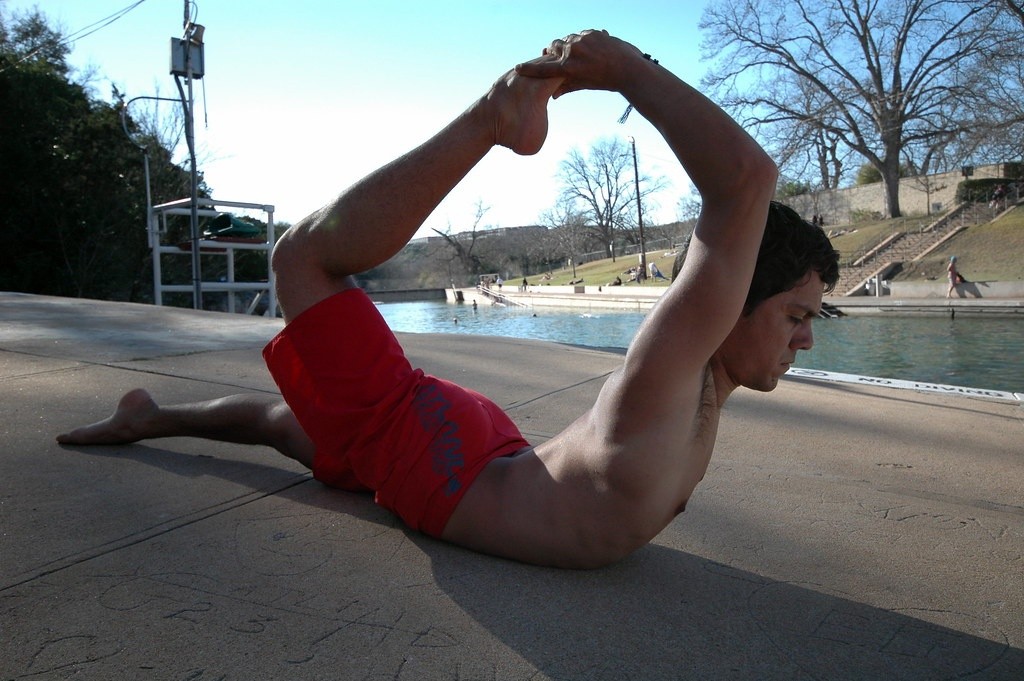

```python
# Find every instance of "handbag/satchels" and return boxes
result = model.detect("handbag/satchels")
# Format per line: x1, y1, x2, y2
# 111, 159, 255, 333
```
956, 273, 965, 283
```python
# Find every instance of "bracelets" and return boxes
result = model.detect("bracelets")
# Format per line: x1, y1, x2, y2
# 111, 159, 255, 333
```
617, 53, 659, 124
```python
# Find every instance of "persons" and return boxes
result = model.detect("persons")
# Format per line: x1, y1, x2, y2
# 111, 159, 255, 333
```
946, 256, 959, 299
55, 29, 840, 570
811, 215, 825, 228
453, 261, 657, 324
993, 184, 1004, 212
